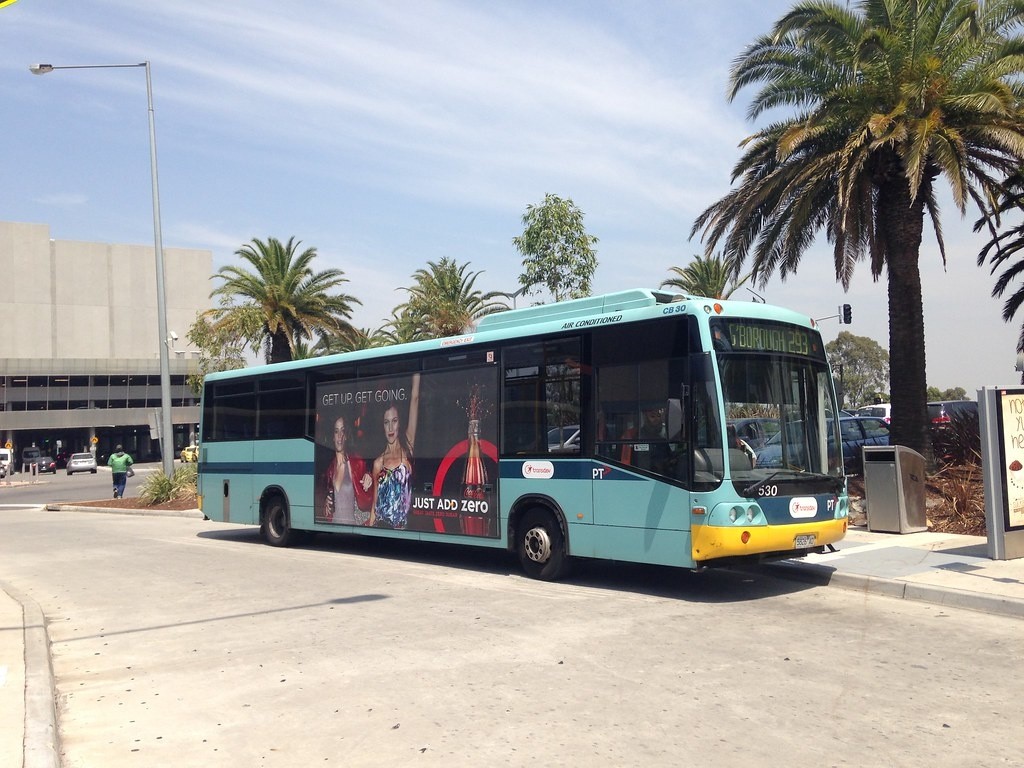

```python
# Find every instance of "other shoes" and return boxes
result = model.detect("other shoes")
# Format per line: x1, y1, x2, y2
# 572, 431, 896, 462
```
117, 495, 122, 499
114, 490, 118, 498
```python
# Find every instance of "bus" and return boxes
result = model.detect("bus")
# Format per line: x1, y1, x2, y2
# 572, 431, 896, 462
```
200, 288, 852, 577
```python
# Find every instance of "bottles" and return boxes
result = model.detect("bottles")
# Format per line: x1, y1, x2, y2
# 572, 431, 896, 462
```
458, 420, 491, 536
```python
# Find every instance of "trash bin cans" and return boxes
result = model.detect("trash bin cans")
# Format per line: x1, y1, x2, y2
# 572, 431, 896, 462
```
861, 444, 928, 532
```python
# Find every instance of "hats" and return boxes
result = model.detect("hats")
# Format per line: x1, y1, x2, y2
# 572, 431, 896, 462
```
115, 445, 122, 451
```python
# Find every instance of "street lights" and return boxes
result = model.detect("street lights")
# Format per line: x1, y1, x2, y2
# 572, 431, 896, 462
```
24, 58, 176, 493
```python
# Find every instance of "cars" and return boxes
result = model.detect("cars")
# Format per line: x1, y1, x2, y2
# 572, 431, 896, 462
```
835, 402, 977, 473
180, 446, 200, 462
66, 452, 97, 475
0, 448, 15, 477
20, 447, 57, 475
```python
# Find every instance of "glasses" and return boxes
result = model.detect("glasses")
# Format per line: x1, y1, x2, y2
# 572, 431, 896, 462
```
646, 412, 661, 418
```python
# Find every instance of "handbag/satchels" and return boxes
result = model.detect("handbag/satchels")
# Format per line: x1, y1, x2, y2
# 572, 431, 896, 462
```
125, 456, 135, 477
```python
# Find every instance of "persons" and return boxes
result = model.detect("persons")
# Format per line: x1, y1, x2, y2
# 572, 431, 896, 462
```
369, 373, 420, 530
321, 414, 373, 524
629, 396, 682, 482
107, 444, 133, 499
727, 425, 757, 468
